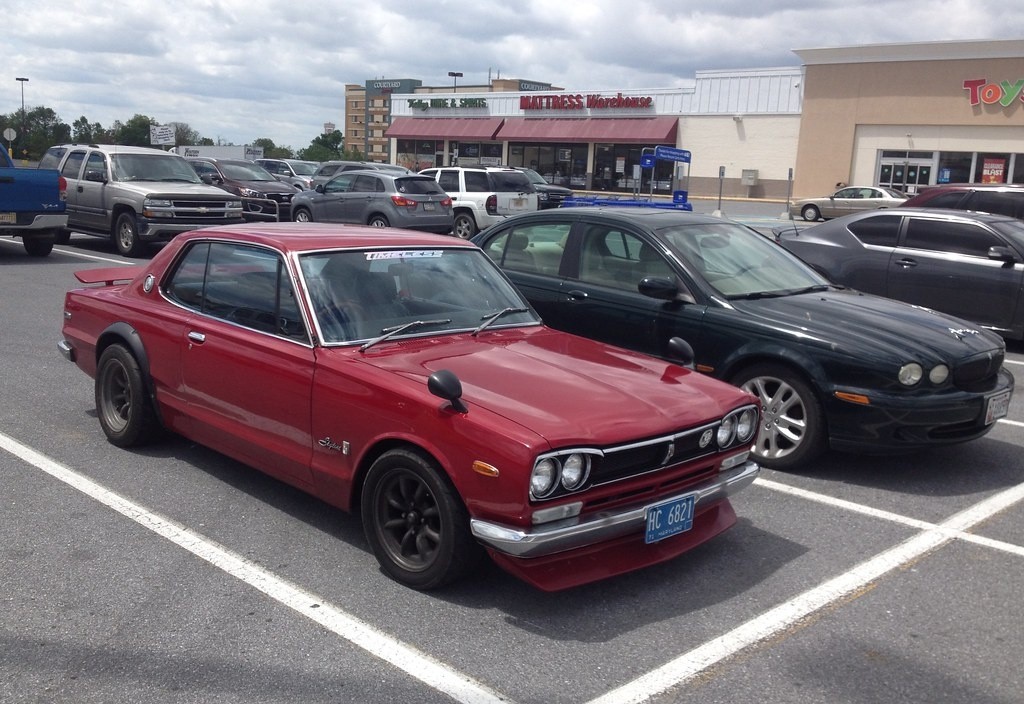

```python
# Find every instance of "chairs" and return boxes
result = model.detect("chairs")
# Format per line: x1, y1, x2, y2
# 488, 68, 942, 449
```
354, 271, 409, 321
633, 243, 678, 286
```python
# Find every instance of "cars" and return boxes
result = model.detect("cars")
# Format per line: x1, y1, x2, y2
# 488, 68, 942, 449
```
57, 221, 761, 593
771, 207, 1024, 340
166, 146, 575, 241
790, 186, 910, 222
896, 182, 1024, 225
398, 204, 1014, 472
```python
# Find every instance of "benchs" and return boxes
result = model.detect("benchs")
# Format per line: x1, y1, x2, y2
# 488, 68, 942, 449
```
492, 231, 570, 275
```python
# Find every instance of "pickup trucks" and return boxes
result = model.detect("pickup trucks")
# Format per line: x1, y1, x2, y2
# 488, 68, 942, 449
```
0, 142, 71, 257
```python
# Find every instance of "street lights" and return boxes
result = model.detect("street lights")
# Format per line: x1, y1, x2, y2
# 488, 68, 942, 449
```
16, 77, 30, 140
448, 72, 463, 93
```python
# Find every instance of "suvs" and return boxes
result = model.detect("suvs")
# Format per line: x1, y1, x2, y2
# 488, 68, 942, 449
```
37, 142, 247, 258
